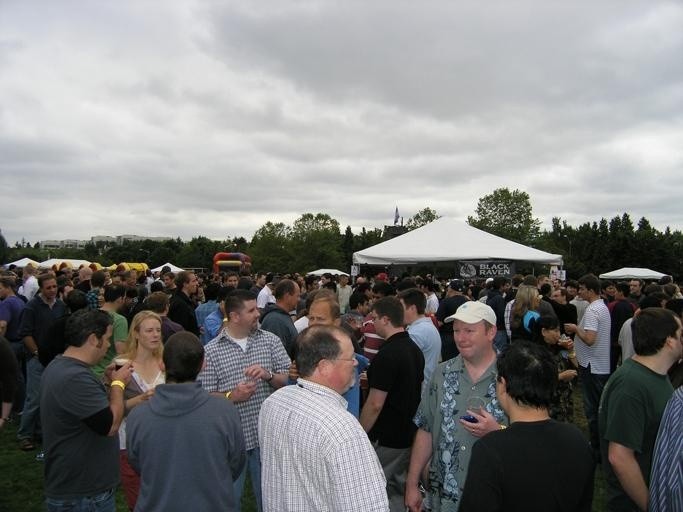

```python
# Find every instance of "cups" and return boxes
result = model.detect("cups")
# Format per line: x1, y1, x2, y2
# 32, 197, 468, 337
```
115, 359, 129, 372
467, 395, 484, 418
559, 334, 569, 349
356, 315, 363, 328
564, 323, 572, 334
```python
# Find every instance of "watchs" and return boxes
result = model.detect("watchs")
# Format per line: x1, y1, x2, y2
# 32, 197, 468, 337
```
2, 416, 10, 423
568, 353, 576, 358
267, 371, 274, 382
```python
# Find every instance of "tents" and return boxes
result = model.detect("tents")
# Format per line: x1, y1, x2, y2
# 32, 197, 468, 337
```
599, 268, 673, 287
352, 217, 563, 284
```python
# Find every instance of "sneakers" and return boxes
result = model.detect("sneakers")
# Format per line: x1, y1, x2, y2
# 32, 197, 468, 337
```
19, 438, 36, 450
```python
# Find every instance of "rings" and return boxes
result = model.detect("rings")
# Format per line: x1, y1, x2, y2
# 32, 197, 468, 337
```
472, 429, 475, 433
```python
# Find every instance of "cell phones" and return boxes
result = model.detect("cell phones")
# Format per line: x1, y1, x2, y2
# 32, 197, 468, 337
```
460, 415, 478, 423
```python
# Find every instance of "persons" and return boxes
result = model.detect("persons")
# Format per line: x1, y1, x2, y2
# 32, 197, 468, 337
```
509, 285, 540, 339
530, 315, 577, 424
126, 332, 246, 512
0, 278, 25, 415
648, 385, 682, 512
564, 281, 579, 298
551, 274, 557, 282
102, 311, 165, 512
204, 286, 237, 340
0, 263, 178, 297
644, 276, 683, 307
608, 281, 638, 373
564, 274, 611, 465
91, 283, 126, 380
486, 278, 507, 352
398, 289, 442, 393
196, 289, 293, 512
337, 274, 352, 314
294, 289, 338, 333
551, 289, 577, 341
435, 280, 470, 363
359, 298, 426, 512
0, 335, 18, 427
459, 338, 594, 512
617, 297, 663, 364
421, 279, 439, 328
404, 301, 508, 511
550, 269, 563, 285
167, 271, 204, 337
194, 270, 397, 291
346, 284, 396, 361
143, 292, 184, 343
666, 299, 683, 390
40, 310, 135, 512
257, 277, 284, 329
599, 307, 682, 511
258, 324, 390, 512
505, 275, 538, 340
259, 279, 299, 359
562, 279, 569, 287
553, 279, 566, 291
16, 274, 66, 450
397, 276, 551, 301
296, 282, 306, 320
286, 298, 359, 421
195, 284, 220, 325
600, 281, 616, 306
342, 293, 369, 325
629, 279, 645, 306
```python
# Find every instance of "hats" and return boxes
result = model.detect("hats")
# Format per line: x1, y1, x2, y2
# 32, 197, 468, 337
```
444, 300, 496, 326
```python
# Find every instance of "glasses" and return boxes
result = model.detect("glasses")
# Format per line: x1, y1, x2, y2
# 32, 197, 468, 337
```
327, 355, 355, 364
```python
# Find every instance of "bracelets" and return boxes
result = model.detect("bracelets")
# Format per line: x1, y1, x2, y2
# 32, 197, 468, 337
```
111, 380, 125, 390
223, 318, 228, 322
225, 391, 231, 398
500, 424, 507, 430
